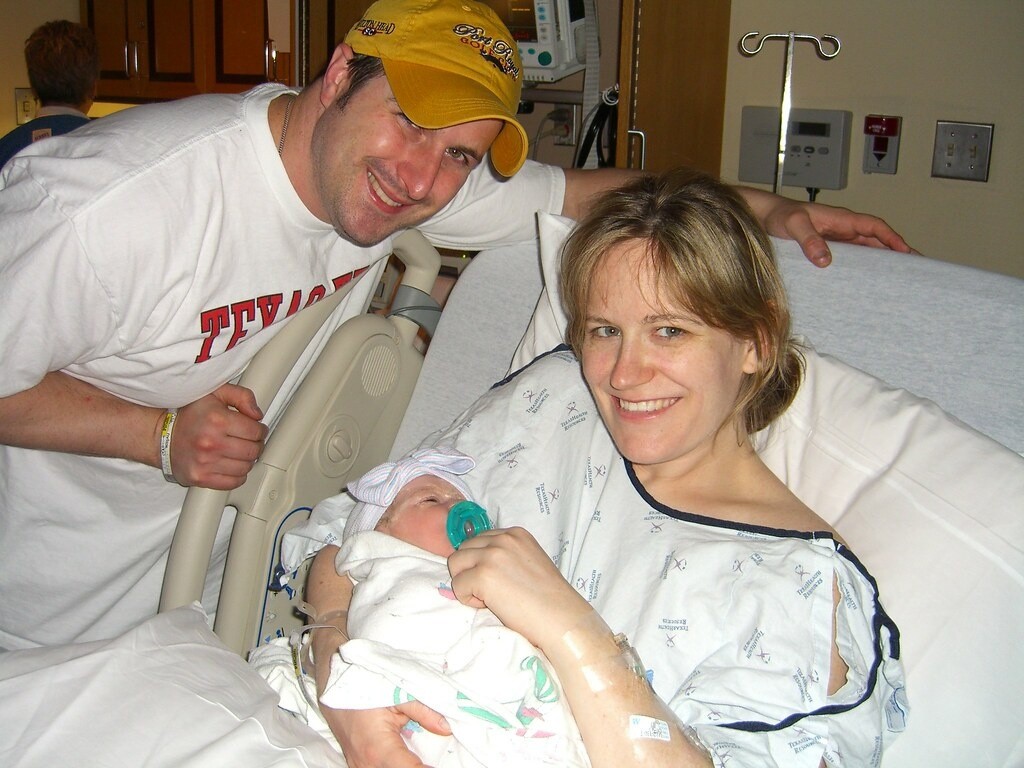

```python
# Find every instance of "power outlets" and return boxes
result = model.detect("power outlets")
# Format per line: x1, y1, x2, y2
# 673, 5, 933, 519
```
14, 88, 35, 125
930, 119, 994, 182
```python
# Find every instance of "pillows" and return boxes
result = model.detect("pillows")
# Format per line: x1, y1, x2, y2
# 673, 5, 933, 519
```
505, 208, 1024, 768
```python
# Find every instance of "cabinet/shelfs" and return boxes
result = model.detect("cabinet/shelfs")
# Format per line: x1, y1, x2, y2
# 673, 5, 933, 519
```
79, 0, 206, 105
306, 0, 731, 181
207, 0, 296, 95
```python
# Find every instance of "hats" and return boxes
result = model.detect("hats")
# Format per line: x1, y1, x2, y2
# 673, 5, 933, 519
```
342, 0, 529, 179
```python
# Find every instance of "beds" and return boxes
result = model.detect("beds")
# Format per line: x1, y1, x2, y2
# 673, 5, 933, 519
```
157, 228, 1024, 768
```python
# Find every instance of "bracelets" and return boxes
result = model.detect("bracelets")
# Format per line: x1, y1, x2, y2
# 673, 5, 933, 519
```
157, 404, 180, 477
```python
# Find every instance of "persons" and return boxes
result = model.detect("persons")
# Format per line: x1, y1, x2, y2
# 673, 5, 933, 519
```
0, 0, 925, 656
306, 171, 890, 768
